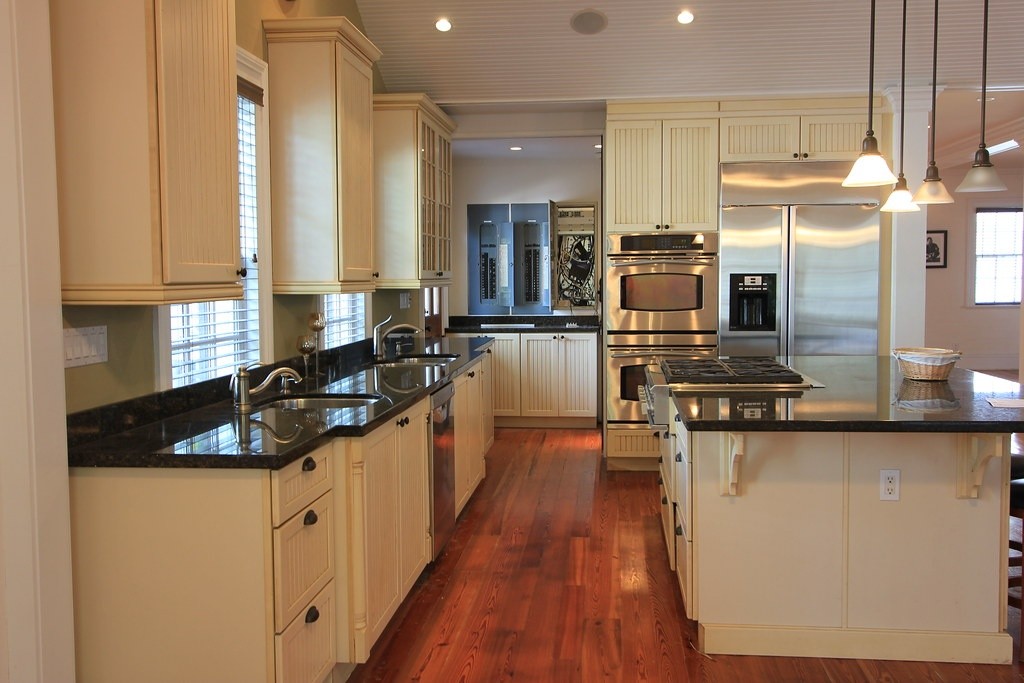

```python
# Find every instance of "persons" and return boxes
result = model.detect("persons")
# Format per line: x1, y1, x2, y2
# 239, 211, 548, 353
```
926, 237, 940, 261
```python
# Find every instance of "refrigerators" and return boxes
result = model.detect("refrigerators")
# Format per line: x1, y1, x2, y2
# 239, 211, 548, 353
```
720, 161, 880, 356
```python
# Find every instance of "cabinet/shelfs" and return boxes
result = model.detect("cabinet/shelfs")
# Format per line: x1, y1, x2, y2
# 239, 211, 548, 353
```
658, 388, 1013, 665
48, 0, 247, 305
69, 349, 495, 683
261, 40, 379, 295
372, 109, 453, 289
606, 114, 883, 236
445, 332, 598, 428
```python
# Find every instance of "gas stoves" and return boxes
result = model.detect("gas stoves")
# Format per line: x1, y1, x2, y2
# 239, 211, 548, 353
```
637, 355, 811, 428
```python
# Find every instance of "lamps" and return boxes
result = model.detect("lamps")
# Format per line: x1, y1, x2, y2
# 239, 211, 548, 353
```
911, 0, 957, 204
953, 0, 1010, 194
879, 0, 920, 212
841, 0, 901, 188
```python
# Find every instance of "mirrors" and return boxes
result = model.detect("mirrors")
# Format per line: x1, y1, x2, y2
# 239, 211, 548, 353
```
447, 136, 603, 318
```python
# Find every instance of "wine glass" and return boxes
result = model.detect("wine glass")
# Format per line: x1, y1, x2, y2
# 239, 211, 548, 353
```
308, 313, 326, 378
296, 335, 316, 381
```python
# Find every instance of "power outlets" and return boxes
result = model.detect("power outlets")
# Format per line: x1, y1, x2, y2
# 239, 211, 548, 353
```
879, 470, 900, 501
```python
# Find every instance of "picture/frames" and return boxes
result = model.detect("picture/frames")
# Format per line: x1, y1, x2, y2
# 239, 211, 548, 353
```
926, 230, 947, 268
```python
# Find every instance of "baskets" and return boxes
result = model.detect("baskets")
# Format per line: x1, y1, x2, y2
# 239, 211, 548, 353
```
890, 376, 961, 413
892, 345, 963, 381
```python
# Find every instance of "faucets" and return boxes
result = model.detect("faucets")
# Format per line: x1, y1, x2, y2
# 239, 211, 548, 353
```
230, 362, 302, 410
373, 314, 424, 356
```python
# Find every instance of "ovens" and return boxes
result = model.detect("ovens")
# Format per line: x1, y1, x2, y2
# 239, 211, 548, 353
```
606, 231, 719, 346
606, 344, 717, 428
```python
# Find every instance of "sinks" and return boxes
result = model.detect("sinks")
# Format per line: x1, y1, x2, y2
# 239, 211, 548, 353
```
258, 398, 380, 408
373, 357, 457, 366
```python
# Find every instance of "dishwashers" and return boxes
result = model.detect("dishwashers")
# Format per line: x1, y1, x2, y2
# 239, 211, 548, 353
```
427, 381, 455, 562
